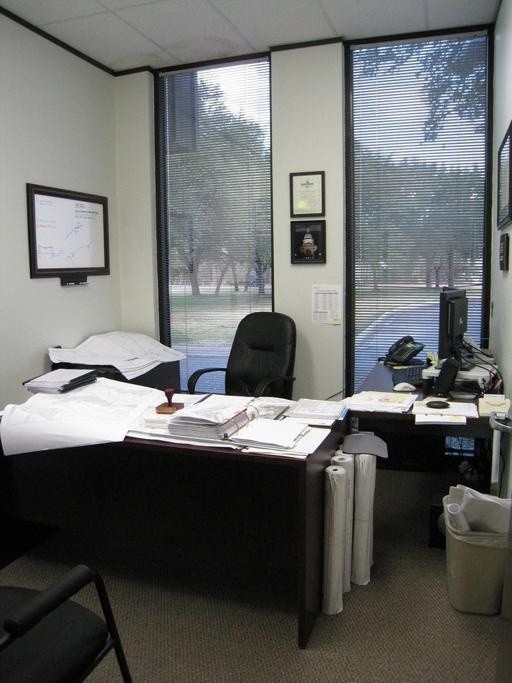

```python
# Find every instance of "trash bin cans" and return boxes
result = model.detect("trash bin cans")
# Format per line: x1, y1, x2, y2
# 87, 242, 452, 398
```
443, 494, 509, 615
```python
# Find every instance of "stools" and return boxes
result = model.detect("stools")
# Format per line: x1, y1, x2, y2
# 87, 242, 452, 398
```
0, 559, 131, 682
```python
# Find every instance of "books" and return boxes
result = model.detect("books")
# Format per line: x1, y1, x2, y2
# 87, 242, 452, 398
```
412, 396, 478, 425
341, 391, 419, 413
478, 394, 510, 416
23, 367, 97, 395
167, 394, 347, 459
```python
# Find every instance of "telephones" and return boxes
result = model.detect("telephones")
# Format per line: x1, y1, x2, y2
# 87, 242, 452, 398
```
389, 337, 425, 364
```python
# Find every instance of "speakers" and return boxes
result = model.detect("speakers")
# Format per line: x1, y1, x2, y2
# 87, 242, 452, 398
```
432, 357, 459, 401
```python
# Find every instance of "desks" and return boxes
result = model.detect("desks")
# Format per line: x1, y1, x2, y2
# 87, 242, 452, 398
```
0, 386, 357, 650
354, 357, 511, 550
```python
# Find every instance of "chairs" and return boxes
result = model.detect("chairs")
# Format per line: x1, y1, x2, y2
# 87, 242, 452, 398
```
187, 312, 296, 398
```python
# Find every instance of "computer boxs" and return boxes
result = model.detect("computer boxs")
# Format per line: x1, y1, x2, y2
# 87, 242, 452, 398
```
440, 348, 496, 364
421, 364, 499, 389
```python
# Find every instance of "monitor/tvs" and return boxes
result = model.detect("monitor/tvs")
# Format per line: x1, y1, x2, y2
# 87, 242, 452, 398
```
434, 286, 475, 371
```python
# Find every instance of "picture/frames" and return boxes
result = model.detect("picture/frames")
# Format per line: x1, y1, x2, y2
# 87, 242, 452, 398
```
497, 121, 511, 230
290, 219, 327, 264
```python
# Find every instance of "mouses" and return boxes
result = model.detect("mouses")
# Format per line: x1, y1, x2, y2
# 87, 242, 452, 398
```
392, 382, 417, 392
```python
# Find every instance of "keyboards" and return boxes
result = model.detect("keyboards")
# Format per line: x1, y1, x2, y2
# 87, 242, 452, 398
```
406, 367, 424, 388
